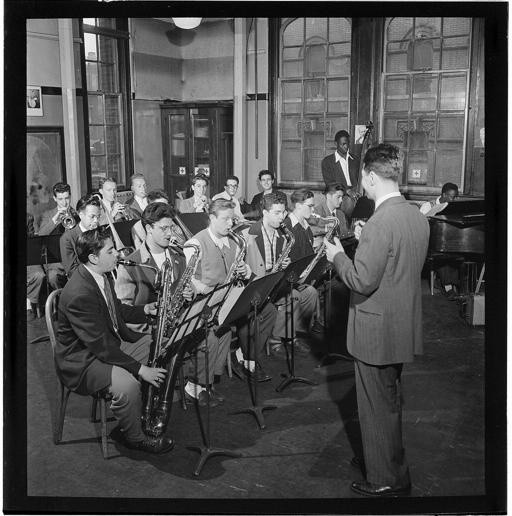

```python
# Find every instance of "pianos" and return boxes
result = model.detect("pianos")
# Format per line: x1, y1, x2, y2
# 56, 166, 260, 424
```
429, 196, 487, 297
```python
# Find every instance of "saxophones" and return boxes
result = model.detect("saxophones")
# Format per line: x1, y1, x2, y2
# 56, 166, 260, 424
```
222, 227, 244, 300
118, 259, 206, 435
162, 238, 199, 336
269, 222, 295, 274
299, 213, 339, 287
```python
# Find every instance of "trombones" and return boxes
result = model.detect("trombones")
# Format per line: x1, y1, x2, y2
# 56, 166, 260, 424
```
92, 194, 134, 260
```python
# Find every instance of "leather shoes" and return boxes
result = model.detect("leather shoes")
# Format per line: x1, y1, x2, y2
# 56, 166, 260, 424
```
184, 385, 226, 408
230, 350, 272, 383
27, 309, 35, 321
110, 425, 175, 453
445, 288, 458, 300
287, 338, 311, 353
351, 477, 411, 496
270, 346, 291, 360
313, 320, 326, 332
353, 454, 366, 470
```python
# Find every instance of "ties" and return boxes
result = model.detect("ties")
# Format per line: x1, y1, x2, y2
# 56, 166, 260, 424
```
104, 278, 119, 330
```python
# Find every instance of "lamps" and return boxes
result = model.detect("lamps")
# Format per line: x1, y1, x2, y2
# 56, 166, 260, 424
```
172, 17, 202, 29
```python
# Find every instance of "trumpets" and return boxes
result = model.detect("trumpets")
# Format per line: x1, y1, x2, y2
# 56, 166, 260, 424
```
61, 209, 75, 229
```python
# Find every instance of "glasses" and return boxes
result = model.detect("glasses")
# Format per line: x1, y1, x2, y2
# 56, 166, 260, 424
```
227, 184, 238, 188
153, 223, 174, 232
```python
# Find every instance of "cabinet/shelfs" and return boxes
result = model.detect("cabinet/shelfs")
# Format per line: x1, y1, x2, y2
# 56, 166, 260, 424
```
159, 100, 233, 203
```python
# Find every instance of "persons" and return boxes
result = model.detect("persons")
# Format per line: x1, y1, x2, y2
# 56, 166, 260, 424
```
321, 129, 360, 191
27, 172, 348, 455
323, 143, 433, 499
419, 182, 464, 301
